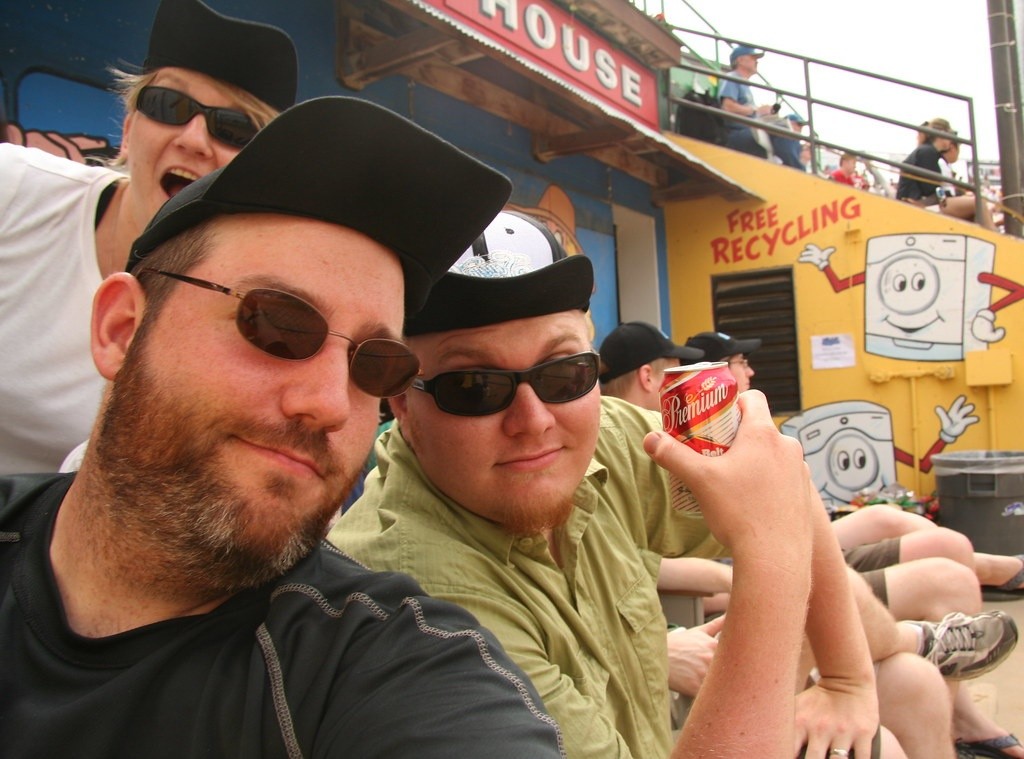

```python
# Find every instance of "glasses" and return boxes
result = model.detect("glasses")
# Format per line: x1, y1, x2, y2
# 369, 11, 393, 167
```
136, 85, 258, 148
133, 268, 421, 399
728, 359, 748, 367
410, 350, 600, 416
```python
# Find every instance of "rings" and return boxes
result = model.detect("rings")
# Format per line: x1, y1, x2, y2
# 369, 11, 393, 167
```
830, 750, 848, 756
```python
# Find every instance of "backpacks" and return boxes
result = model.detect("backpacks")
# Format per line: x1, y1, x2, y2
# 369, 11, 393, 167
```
674, 90, 728, 145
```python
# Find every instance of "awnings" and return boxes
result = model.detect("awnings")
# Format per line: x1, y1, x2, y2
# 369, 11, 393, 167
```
411, 1, 767, 204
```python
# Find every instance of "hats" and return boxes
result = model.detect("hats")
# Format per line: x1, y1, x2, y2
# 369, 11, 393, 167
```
144, 0, 298, 112
403, 210, 595, 337
785, 114, 809, 126
124, 97, 514, 317
679, 331, 762, 366
597, 321, 706, 383
729, 46, 765, 62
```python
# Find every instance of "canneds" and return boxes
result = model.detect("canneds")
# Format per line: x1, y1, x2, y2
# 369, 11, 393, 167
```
659, 362, 743, 520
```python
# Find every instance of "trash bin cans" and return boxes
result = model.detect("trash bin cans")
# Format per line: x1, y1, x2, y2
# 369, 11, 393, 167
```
930, 451, 1024, 592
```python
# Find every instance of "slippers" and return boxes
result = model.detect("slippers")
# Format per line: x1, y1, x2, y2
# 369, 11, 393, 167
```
980, 555, 1024, 595
954, 733, 1024, 759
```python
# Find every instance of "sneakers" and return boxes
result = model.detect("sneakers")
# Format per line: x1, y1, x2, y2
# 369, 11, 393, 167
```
895, 610, 1018, 681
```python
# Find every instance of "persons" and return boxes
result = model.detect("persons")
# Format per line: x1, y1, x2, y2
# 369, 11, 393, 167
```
321, 211, 906, 759
0, 97, 562, 758
0, 1, 300, 471
719, 48, 1005, 233
598, 323, 1023, 759
